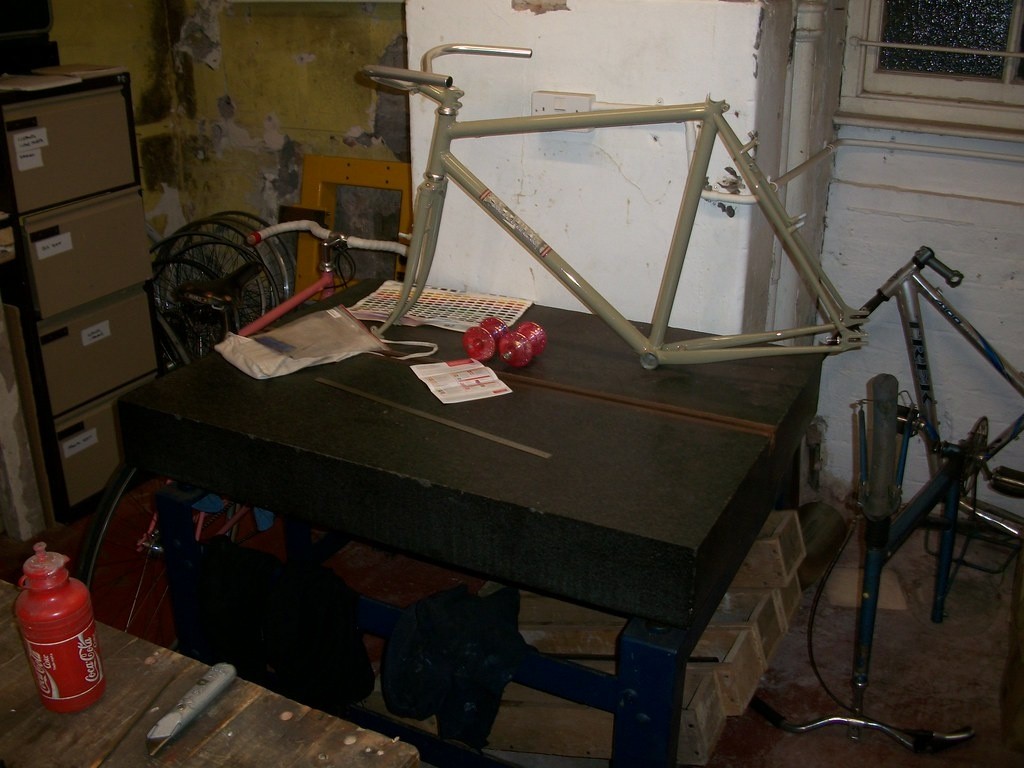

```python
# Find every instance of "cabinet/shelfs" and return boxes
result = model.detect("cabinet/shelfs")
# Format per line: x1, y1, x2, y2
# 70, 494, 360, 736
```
0, 69, 166, 527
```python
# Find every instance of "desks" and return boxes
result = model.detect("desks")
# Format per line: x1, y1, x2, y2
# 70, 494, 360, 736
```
113, 276, 822, 767
0, 581, 421, 768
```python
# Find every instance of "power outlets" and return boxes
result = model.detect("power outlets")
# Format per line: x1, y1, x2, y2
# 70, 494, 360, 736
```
530, 90, 596, 135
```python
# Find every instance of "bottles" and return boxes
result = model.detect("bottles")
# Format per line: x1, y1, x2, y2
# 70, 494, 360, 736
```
14, 543, 106, 711
